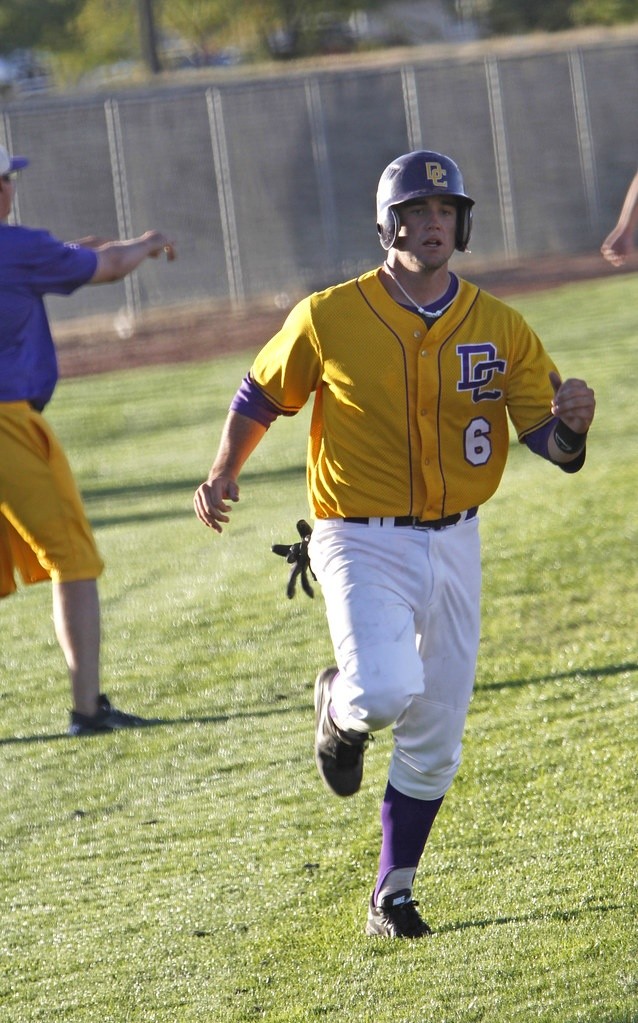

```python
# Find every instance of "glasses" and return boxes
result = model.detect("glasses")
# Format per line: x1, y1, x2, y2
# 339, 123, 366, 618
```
0, 171, 18, 181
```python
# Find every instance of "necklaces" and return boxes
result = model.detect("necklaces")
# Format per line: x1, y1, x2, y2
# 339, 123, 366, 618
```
383, 259, 461, 318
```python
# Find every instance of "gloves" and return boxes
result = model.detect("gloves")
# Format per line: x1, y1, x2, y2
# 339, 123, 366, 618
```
271, 520, 317, 599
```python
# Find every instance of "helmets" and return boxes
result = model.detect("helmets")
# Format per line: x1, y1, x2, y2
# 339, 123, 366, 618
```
375, 150, 475, 253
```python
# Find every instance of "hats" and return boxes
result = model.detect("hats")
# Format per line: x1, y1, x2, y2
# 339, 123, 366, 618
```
0, 147, 28, 178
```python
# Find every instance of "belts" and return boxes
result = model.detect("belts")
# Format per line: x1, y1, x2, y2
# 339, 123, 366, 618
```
344, 506, 479, 530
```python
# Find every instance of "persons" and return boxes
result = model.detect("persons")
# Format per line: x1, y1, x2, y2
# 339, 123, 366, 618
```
1, 147, 175, 737
193, 151, 595, 939
601, 169, 638, 273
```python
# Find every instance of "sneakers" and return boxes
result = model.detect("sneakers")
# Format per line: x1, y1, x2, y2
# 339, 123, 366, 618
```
68, 694, 149, 737
314, 667, 374, 797
366, 888, 432, 939
